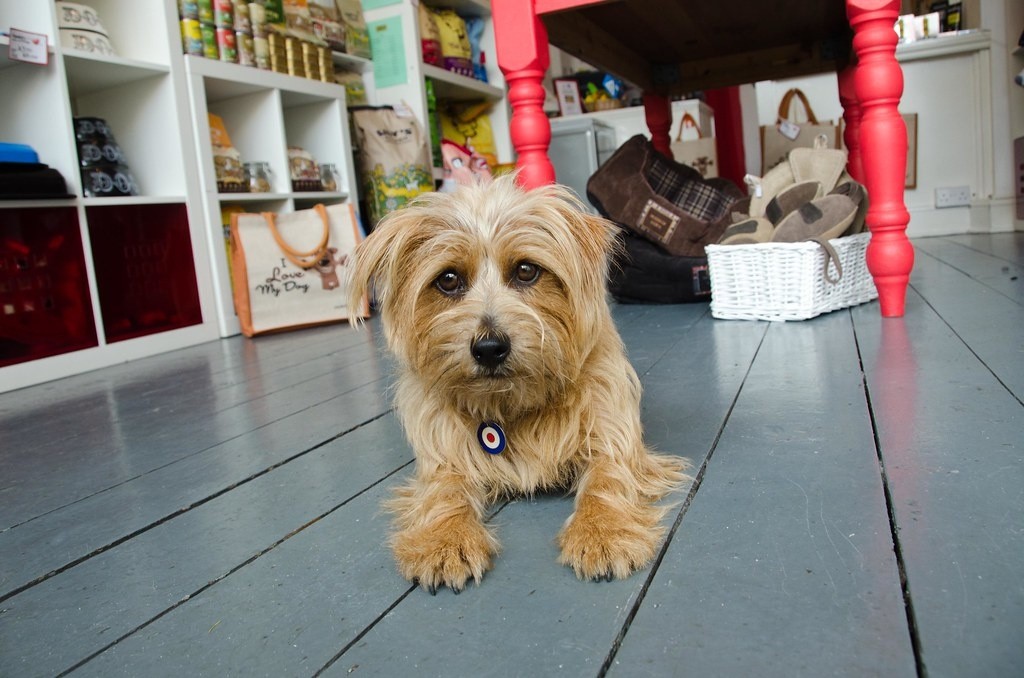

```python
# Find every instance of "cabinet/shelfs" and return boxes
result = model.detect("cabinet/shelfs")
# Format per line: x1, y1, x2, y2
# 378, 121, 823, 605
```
0, 0, 220, 393
183, 53, 375, 339
357, 0, 519, 235
545, 116, 617, 218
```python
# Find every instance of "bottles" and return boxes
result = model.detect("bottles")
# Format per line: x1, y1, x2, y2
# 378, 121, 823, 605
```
930, 0, 961, 31
317, 164, 336, 190
244, 160, 270, 193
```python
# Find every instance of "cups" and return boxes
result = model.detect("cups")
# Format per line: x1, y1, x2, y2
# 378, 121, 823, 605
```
894, 14, 916, 43
915, 12, 939, 39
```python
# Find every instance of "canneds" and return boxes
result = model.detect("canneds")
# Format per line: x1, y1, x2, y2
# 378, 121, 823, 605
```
178, 0, 337, 84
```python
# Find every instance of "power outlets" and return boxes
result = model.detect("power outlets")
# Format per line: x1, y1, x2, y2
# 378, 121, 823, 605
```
935, 186, 971, 209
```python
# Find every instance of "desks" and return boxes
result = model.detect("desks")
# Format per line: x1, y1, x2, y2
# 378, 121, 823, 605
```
489, 0, 915, 318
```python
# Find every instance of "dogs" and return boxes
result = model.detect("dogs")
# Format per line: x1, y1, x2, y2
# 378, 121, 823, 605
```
340, 165, 697, 595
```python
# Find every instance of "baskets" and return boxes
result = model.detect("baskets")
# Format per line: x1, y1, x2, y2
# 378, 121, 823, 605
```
705, 232, 880, 322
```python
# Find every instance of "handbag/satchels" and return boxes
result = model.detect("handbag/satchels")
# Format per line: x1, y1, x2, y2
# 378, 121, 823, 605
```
760, 88, 842, 177
670, 113, 718, 179
229, 203, 370, 338
